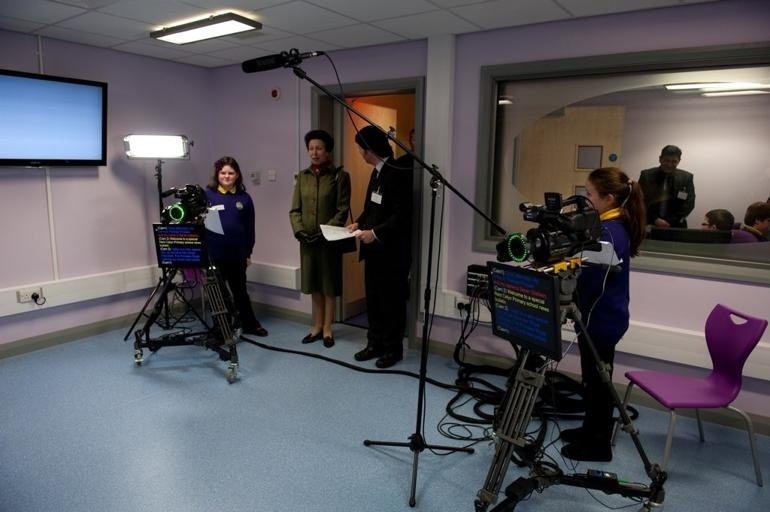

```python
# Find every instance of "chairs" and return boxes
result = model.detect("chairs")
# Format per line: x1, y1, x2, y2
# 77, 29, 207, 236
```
730, 229, 764, 244
608, 300, 769, 493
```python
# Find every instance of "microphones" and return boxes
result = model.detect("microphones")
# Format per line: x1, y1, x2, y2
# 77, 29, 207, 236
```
241, 48, 320, 74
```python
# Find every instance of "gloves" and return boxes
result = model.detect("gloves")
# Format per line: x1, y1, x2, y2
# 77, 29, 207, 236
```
297, 231, 325, 245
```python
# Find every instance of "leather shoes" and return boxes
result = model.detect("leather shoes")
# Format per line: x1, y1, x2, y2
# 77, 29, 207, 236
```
253, 327, 270, 336
324, 335, 334, 347
302, 330, 323, 343
355, 345, 403, 367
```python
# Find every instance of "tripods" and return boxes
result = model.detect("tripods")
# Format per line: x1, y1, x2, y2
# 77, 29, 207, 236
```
124, 161, 213, 343
294, 68, 505, 507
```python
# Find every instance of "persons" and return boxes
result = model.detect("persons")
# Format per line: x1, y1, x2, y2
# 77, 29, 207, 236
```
742, 200, 770, 242
560, 168, 647, 460
200, 156, 268, 336
345, 123, 411, 369
288, 130, 352, 347
702, 208, 735, 230
639, 145, 696, 230
396, 128, 416, 163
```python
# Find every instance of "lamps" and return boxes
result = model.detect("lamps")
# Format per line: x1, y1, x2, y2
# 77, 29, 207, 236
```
147, 12, 264, 47
120, 129, 211, 342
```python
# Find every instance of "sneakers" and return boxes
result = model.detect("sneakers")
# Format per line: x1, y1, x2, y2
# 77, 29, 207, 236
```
560, 429, 612, 461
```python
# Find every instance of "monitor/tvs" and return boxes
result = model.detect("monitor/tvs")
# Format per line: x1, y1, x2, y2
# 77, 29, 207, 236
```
0, 68, 108, 166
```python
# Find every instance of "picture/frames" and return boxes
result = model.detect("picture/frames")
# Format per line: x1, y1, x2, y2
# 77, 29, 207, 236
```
573, 143, 605, 172
574, 185, 588, 197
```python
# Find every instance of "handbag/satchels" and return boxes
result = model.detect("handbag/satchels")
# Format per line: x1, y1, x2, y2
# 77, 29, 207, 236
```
324, 236, 357, 253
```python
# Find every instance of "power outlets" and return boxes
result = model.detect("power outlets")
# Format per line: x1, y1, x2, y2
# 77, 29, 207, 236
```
18, 287, 43, 303
454, 296, 476, 314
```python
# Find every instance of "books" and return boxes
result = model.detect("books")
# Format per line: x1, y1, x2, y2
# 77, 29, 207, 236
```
320, 225, 362, 241
